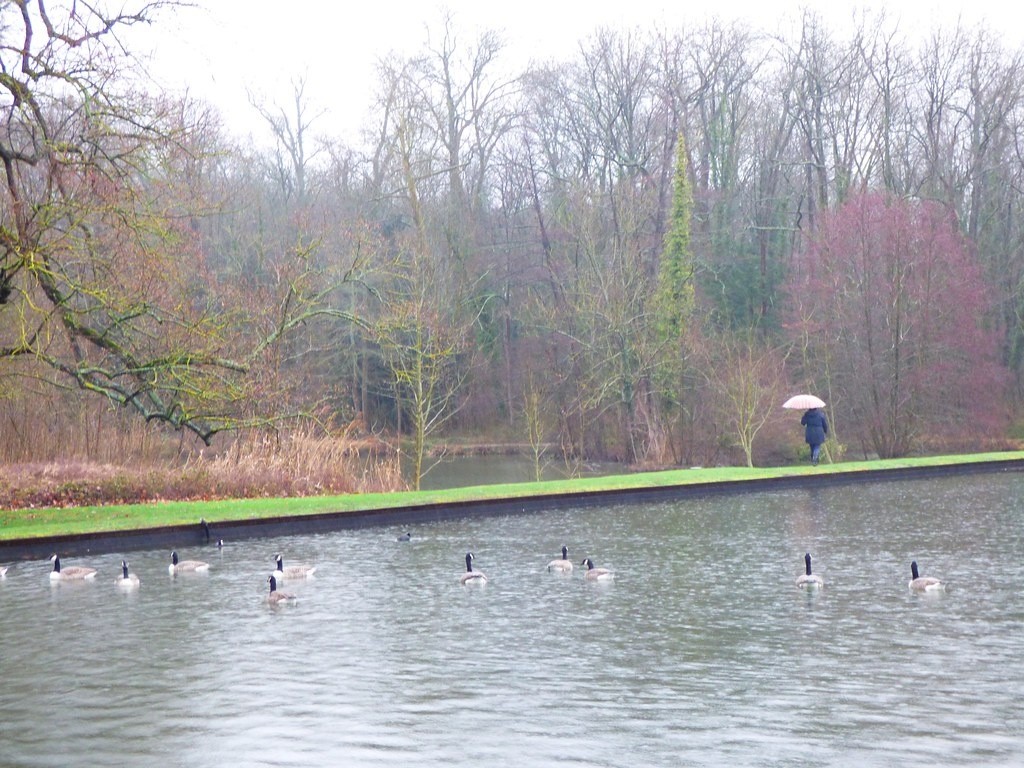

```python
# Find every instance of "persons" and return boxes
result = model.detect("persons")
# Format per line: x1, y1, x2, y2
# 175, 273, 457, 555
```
801, 409, 828, 466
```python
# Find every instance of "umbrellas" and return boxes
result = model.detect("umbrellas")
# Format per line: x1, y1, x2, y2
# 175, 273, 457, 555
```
782, 394, 826, 409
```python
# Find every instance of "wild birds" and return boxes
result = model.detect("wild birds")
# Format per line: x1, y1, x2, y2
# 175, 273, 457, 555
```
461, 552, 488, 585
266, 575, 297, 603
908, 560, 950, 590
114, 559, 140, 587
546, 545, 573, 573
796, 553, 824, 588
45, 552, 98, 581
580, 558, 615, 580
168, 551, 209, 575
215, 536, 224, 546
272, 554, 318, 579
396, 533, 411, 541
